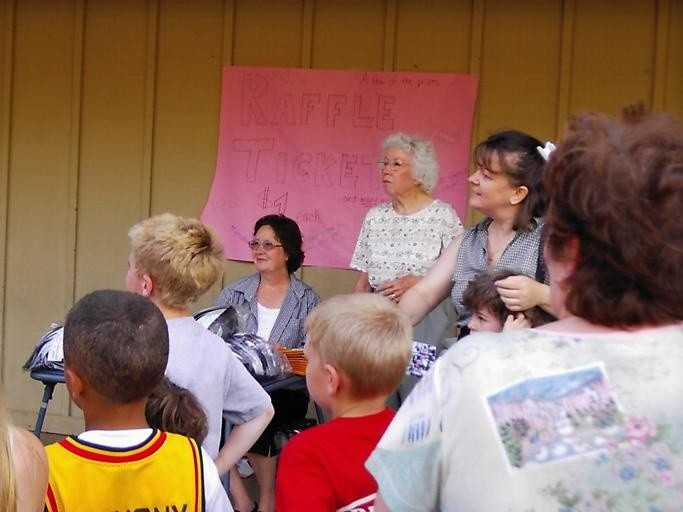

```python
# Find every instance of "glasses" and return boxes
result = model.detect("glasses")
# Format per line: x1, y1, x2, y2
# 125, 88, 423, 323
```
247, 241, 283, 252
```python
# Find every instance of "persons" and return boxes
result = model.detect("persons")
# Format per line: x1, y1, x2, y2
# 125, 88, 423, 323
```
0, 383, 49, 512
273, 292, 412, 512
213, 213, 323, 512
349, 132, 465, 420
364, 108, 681, 512
143, 375, 208, 444
123, 212, 275, 496
38, 289, 235, 512
460, 267, 543, 333
399, 127, 563, 343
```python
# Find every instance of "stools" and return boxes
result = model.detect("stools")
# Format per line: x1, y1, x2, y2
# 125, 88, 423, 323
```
28, 367, 67, 438
216, 372, 326, 498
